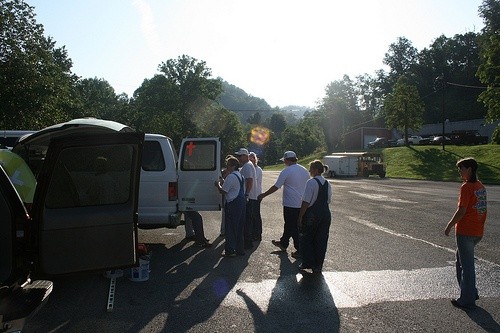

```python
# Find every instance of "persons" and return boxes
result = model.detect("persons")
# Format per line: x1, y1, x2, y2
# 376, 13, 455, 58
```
445, 157, 487, 309
297, 159, 332, 270
183, 159, 210, 243
257, 151, 311, 259
214, 147, 262, 257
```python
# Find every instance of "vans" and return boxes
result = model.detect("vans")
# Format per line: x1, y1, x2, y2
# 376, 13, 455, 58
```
0, 130, 222, 229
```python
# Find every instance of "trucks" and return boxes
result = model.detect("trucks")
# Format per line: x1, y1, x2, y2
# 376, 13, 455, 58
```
323, 152, 386, 179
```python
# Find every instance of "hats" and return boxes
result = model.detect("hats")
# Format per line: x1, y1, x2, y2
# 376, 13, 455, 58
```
280, 151, 296, 160
234, 148, 248, 155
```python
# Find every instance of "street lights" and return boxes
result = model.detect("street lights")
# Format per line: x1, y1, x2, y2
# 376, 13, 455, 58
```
442, 118, 449, 150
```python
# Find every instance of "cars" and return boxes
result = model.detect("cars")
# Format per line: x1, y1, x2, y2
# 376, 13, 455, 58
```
368, 130, 489, 149
0, 119, 145, 333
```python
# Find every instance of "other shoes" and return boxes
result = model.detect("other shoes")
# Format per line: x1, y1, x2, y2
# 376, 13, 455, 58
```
298, 263, 313, 269
272, 240, 287, 252
451, 299, 471, 309
186, 234, 195, 240
291, 251, 303, 260
196, 239, 210, 246
193, 232, 195, 234
457, 294, 480, 302
